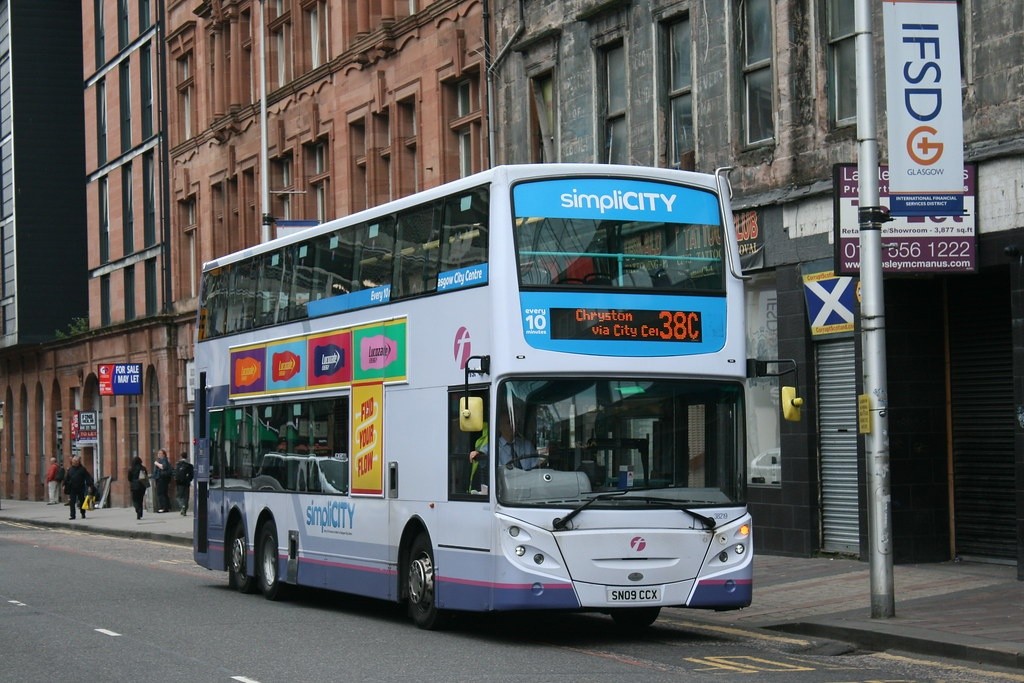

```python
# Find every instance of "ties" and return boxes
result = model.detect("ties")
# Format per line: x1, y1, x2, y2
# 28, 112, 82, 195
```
507, 442, 522, 470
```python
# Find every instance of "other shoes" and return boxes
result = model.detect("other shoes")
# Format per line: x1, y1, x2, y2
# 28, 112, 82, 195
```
181, 506, 187, 516
158, 509, 169, 513
82, 514, 85, 518
64, 502, 71, 506
137, 512, 142, 519
69, 516, 76, 520
47, 502, 58, 505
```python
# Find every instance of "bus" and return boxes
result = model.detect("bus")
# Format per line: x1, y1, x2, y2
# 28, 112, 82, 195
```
191, 161, 807, 632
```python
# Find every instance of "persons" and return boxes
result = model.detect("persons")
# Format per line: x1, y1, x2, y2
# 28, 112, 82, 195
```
127, 456, 147, 519
61, 454, 94, 520
45, 457, 60, 504
174, 452, 193, 516
468, 412, 544, 471
152, 449, 171, 512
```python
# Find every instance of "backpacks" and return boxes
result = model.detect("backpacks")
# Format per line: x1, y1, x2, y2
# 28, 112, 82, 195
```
53, 464, 65, 482
177, 460, 192, 486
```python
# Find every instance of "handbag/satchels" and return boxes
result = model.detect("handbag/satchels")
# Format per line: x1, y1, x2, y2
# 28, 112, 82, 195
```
81, 493, 95, 511
138, 466, 151, 488
159, 458, 173, 479
